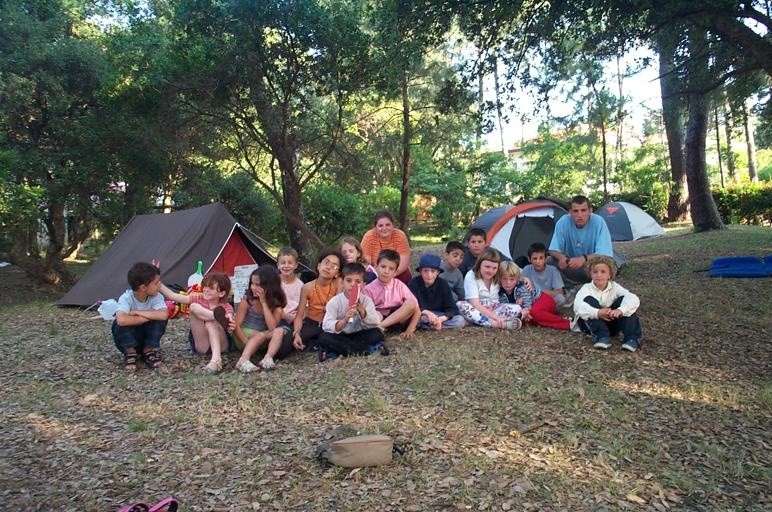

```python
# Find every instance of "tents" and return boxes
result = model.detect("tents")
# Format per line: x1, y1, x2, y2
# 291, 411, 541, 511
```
462, 197, 628, 269
593, 201, 666, 241
51, 203, 278, 307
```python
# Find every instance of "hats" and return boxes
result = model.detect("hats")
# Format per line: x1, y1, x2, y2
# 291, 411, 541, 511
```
416, 254, 444, 273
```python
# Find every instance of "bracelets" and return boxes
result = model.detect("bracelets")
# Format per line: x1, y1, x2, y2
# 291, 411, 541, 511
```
583, 255, 588, 262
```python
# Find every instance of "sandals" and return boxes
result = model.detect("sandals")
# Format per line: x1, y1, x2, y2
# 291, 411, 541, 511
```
202, 360, 225, 374
319, 345, 333, 362
124, 350, 137, 372
141, 347, 161, 368
214, 306, 230, 330
368, 341, 389, 355
117, 497, 179, 512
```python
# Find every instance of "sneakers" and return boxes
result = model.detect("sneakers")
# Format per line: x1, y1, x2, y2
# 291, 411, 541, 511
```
594, 337, 612, 349
622, 335, 641, 351
562, 287, 576, 307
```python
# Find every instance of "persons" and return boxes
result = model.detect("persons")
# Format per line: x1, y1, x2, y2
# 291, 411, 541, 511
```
568, 255, 641, 352
111, 263, 171, 370
153, 236, 421, 374
498, 259, 571, 331
521, 242, 567, 310
458, 228, 510, 277
437, 241, 464, 300
455, 248, 522, 331
547, 194, 614, 308
360, 211, 412, 285
407, 254, 465, 331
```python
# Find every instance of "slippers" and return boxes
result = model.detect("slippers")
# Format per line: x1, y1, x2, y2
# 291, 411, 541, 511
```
257, 356, 278, 369
504, 318, 522, 330
236, 361, 261, 374
